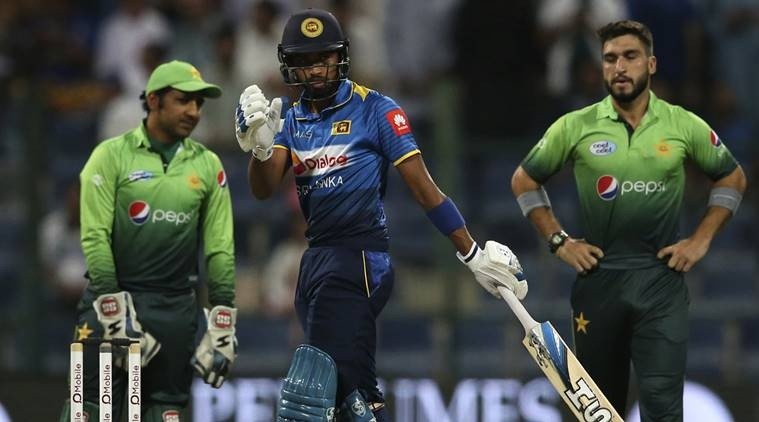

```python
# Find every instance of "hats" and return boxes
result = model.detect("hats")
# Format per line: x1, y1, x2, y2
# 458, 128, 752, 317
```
145, 60, 222, 100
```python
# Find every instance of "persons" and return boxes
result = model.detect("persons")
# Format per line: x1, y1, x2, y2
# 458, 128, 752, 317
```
62, 60, 239, 422
511, 21, 745, 422
38, 0, 388, 309
236, 12, 528, 422
537, 0, 627, 105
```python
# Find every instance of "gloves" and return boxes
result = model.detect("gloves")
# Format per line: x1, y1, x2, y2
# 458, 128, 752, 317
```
192, 305, 238, 388
456, 240, 529, 301
91, 290, 161, 370
234, 84, 286, 160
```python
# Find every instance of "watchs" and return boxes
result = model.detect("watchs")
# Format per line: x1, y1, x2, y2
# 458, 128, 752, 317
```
549, 231, 568, 254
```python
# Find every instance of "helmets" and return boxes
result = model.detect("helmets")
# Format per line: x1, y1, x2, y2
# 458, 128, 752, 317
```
279, 9, 349, 101
